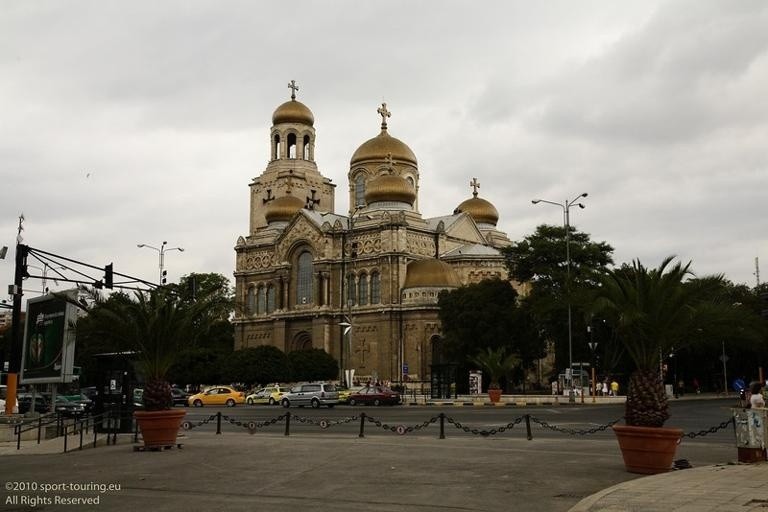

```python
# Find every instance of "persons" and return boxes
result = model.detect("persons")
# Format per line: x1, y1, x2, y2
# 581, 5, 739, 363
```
596, 378, 619, 397
749, 383, 765, 409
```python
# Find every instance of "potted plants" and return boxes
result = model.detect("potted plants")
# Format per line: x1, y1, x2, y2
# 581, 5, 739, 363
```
71, 271, 256, 455
581, 254, 729, 475
463, 342, 525, 403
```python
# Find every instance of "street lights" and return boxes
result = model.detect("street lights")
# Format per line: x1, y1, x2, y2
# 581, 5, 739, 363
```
136, 232, 185, 411
531, 192, 589, 401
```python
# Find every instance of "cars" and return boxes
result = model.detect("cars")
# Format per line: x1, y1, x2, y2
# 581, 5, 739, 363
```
187, 383, 401, 408
0, 384, 93, 417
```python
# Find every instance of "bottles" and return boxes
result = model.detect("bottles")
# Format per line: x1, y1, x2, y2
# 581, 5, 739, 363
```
29, 313, 46, 368
